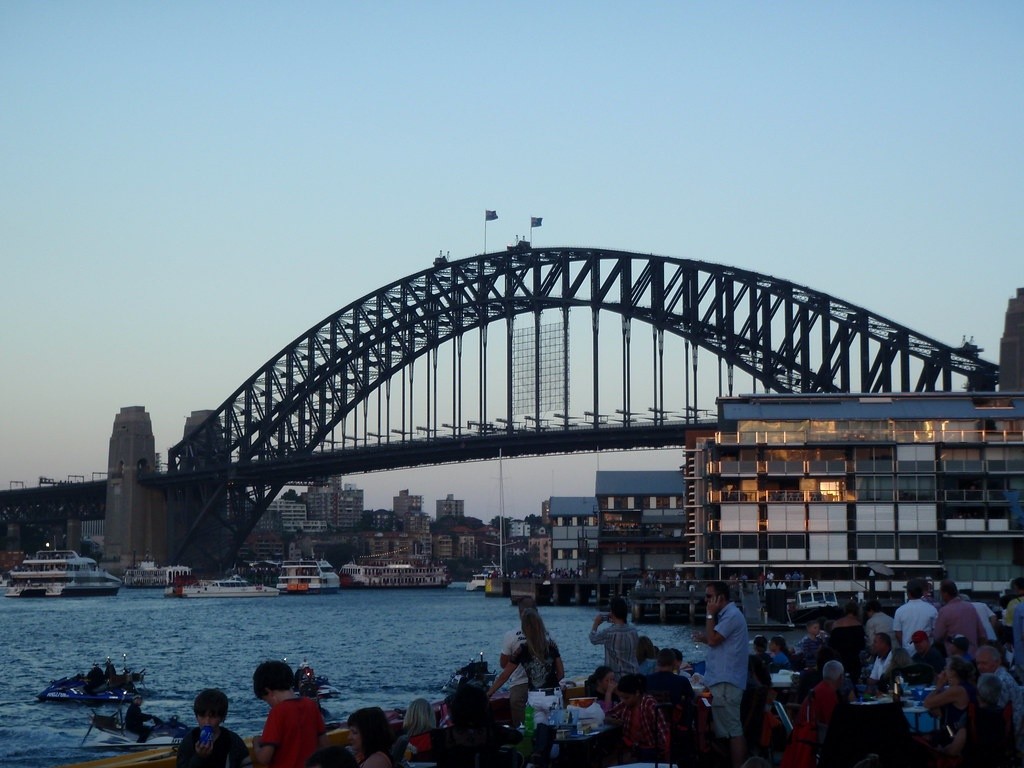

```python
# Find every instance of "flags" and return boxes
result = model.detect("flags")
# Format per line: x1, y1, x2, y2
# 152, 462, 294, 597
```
531, 217, 543, 227
486, 211, 498, 221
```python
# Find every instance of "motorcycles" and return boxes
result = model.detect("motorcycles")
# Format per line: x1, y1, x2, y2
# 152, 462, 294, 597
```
291, 667, 341, 699
87, 710, 191, 751
36, 673, 142, 705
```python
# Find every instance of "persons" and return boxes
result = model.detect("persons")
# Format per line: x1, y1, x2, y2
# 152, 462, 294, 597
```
347, 707, 395, 768
304, 746, 360, 768
721, 484, 738, 500
176, 689, 252, 768
124, 695, 153, 744
741, 577, 1024, 768
297, 679, 320, 714
691, 582, 749, 768
757, 570, 801, 592
742, 573, 747, 592
10, 553, 81, 574
251, 661, 324, 768
83, 664, 127, 694
396, 597, 565, 768
729, 573, 738, 591
488, 567, 579, 581
638, 570, 696, 592
588, 597, 699, 768
605, 523, 661, 536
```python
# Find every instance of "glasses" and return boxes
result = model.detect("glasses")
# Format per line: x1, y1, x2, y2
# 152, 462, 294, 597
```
704, 593, 716, 599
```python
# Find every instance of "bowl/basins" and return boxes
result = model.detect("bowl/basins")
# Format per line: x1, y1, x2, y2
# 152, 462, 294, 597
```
569, 697, 598, 708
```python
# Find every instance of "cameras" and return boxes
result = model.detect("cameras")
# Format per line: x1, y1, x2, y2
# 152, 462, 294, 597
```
601, 615, 609, 621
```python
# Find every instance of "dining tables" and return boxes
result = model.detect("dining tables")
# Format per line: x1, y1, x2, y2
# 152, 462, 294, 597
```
846, 688, 942, 733
519, 723, 624, 768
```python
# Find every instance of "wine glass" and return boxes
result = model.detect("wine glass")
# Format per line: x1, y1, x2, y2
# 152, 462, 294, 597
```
690, 631, 704, 653
910, 689, 931, 706
856, 684, 867, 703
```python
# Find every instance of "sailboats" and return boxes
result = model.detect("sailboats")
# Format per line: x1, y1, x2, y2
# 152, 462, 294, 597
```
466, 448, 510, 591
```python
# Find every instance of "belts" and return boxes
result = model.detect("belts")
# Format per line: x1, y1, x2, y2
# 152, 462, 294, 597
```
529, 687, 562, 692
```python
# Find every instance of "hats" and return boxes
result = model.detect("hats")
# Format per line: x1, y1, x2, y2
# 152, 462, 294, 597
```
909, 631, 928, 645
945, 633, 969, 651
863, 601, 881, 613
903, 579, 923, 591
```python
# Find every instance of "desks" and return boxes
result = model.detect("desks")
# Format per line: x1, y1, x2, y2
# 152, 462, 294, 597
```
770, 673, 800, 687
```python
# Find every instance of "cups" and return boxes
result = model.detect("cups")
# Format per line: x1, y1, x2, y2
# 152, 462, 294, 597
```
556, 730, 571, 740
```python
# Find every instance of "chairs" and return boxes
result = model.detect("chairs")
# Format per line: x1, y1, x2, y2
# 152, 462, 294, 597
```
770, 699, 793, 734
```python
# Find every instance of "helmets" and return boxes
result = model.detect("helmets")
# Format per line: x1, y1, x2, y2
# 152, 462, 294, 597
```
299, 662, 312, 674
130, 693, 142, 700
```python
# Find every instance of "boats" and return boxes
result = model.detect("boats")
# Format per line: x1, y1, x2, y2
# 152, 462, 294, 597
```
338, 551, 452, 589
786, 578, 839, 621
124, 554, 192, 589
5, 536, 125, 598
277, 558, 340, 594
442, 650, 511, 696
163, 570, 281, 598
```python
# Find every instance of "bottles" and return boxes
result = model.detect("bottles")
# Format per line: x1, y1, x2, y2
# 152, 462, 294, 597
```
893, 675, 903, 703
525, 701, 534, 733
555, 698, 562, 712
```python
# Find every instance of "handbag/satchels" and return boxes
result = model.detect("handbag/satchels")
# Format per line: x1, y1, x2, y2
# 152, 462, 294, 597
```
761, 701, 782, 747
567, 702, 606, 727
778, 688, 817, 767
